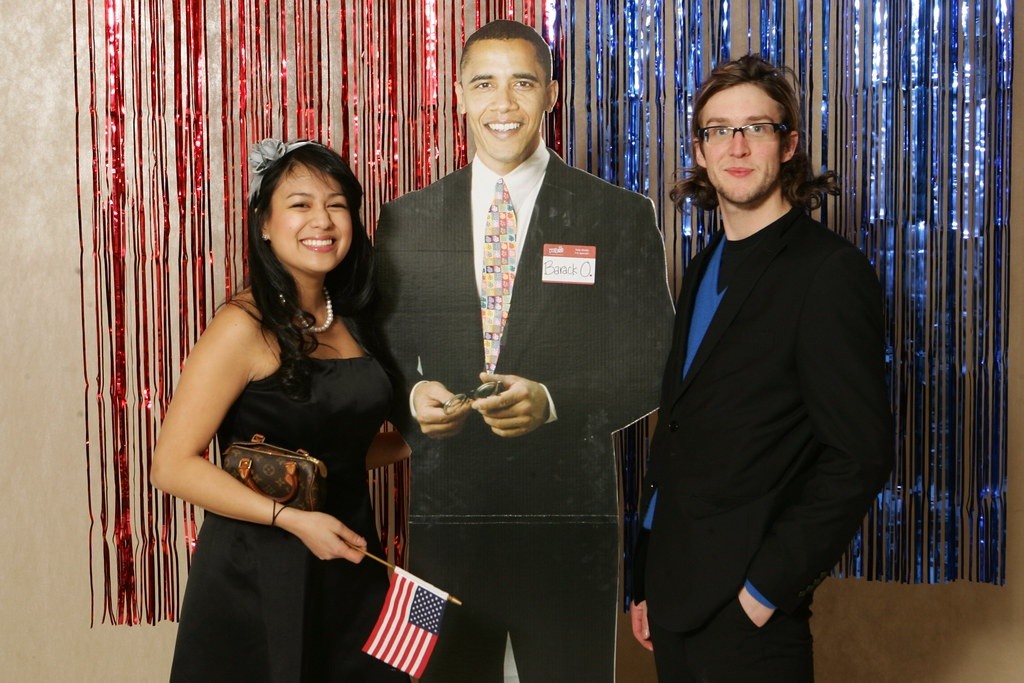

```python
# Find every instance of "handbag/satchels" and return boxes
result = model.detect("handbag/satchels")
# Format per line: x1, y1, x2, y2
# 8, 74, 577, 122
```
224, 434, 328, 512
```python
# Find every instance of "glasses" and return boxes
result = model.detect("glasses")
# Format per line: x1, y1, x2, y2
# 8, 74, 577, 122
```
698, 123, 788, 145
443, 381, 504, 415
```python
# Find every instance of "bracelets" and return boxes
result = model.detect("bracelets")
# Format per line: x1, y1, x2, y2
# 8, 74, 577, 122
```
271, 500, 288, 526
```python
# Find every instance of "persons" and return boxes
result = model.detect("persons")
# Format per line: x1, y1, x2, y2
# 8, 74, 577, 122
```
145, 139, 408, 683
631, 53, 895, 683
356, 18, 677, 683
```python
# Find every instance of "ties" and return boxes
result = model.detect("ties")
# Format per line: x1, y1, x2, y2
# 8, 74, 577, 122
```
480, 177, 519, 375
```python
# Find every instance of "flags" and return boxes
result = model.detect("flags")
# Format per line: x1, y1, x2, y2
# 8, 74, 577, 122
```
361, 566, 450, 679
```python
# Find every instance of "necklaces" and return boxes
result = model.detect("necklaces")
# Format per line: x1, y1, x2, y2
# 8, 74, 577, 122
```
280, 286, 333, 333
